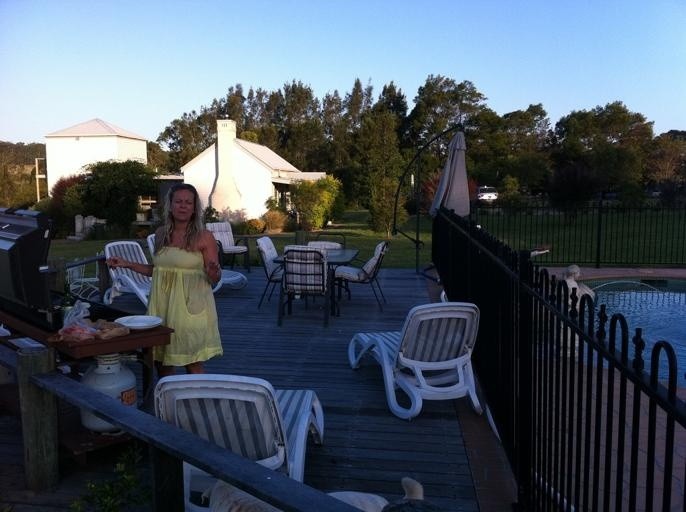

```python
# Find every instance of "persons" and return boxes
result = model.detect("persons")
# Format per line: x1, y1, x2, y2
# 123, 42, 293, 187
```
564, 265, 580, 305
104, 182, 222, 380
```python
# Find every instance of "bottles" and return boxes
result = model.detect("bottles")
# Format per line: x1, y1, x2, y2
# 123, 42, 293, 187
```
60, 284, 76, 313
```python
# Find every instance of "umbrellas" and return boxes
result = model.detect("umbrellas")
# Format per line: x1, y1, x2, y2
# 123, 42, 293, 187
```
428, 131, 471, 220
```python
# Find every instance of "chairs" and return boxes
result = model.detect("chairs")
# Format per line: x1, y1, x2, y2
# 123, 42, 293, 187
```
255, 233, 390, 328
103, 217, 248, 307
349, 303, 485, 418
155, 371, 325, 510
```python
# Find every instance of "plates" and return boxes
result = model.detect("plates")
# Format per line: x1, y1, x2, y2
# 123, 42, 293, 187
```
115, 314, 162, 330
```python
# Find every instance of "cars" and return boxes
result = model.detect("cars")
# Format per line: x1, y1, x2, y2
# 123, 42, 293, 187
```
477, 185, 499, 208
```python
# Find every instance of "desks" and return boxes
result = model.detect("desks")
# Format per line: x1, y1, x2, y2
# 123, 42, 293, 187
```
0, 293, 175, 415
